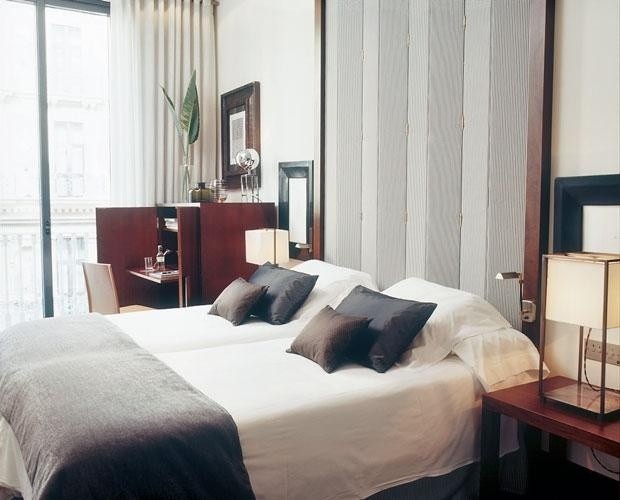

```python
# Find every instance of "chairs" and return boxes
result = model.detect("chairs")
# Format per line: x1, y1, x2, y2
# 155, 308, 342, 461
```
82, 262, 157, 314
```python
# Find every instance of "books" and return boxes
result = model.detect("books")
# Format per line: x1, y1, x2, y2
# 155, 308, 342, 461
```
165, 218, 179, 232
150, 270, 180, 279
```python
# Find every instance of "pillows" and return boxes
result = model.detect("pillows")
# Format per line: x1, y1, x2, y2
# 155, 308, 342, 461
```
453, 329, 551, 391
380, 276, 512, 375
285, 259, 370, 321
333, 285, 438, 373
284, 304, 373, 373
246, 261, 318, 325
207, 277, 269, 326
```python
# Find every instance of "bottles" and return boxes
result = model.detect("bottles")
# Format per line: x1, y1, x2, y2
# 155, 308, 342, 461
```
156, 245, 165, 272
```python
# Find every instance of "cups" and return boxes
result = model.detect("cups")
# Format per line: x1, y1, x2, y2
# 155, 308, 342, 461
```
144, 257, 152, 269
188, 188, 211, 202
209, 179, 229, 202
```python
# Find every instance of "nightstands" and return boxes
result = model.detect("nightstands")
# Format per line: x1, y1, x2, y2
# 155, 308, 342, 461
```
481, 376, 620, 500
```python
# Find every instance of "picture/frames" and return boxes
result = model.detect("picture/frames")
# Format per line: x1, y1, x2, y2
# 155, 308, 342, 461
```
220, 81, 262, 190
278, 160, 314, 261
552, 174, 620, 254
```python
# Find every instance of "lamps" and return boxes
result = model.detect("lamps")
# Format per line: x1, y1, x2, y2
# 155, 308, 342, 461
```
539, 251, 620, 420
245, 230, 290, 265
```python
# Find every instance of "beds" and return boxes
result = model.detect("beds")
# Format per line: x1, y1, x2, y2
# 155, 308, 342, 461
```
0, 260, 549, 500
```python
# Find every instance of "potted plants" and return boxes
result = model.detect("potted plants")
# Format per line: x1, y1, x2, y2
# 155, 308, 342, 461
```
158, 69, 200, 202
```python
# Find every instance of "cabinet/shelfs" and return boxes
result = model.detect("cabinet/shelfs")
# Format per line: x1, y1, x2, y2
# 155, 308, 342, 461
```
96, 200, 276, 306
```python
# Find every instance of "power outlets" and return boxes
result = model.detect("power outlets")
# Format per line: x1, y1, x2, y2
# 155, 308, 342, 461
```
583, 339, 620, 368
